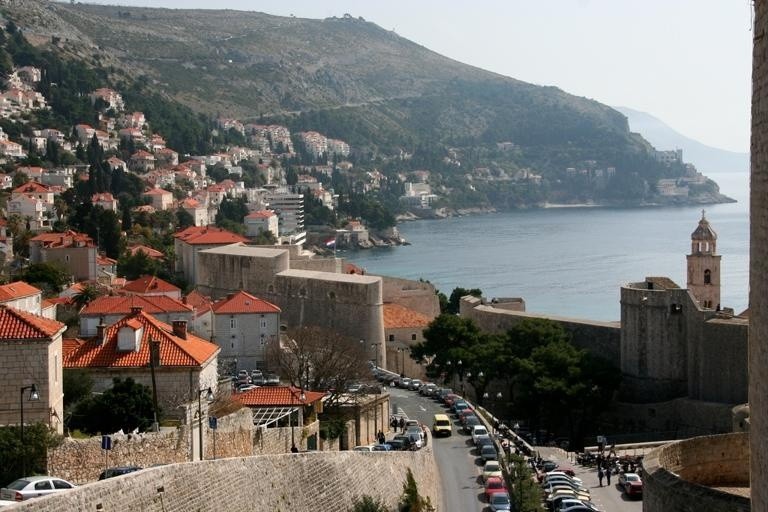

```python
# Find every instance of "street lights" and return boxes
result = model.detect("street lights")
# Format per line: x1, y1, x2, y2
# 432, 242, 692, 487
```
359, 338, 366, 352
396, 346, 410, 379
497, 390, 524, 508
20, 382, 40, 477
95, 226, 101, 254
371, 343, 377, 369
289, 385, 307, 453
193, 386, 215, 460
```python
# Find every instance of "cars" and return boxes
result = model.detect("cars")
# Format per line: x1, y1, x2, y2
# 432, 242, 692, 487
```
617, 472, 642, 486
622, 480, 642, 498
527, 459, 602, 512
483, 459, 511, 512
229, 368, 280, 394
326, 360, 496, 460
0, 475, 78, 502
97, 466, 143, 481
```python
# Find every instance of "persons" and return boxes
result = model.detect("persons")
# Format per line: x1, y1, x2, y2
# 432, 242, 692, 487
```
399, 415, 405, 432
291, 442, 298, 453
393, 417, 398, 433
377, 429, 382, 444
597, 467, 605, 487
607, 437, 617, 456
380, 431, 386, 444
605, 468, 612, 486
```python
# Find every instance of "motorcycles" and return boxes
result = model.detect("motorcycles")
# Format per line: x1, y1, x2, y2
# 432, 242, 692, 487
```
576, 449, 644, 475
